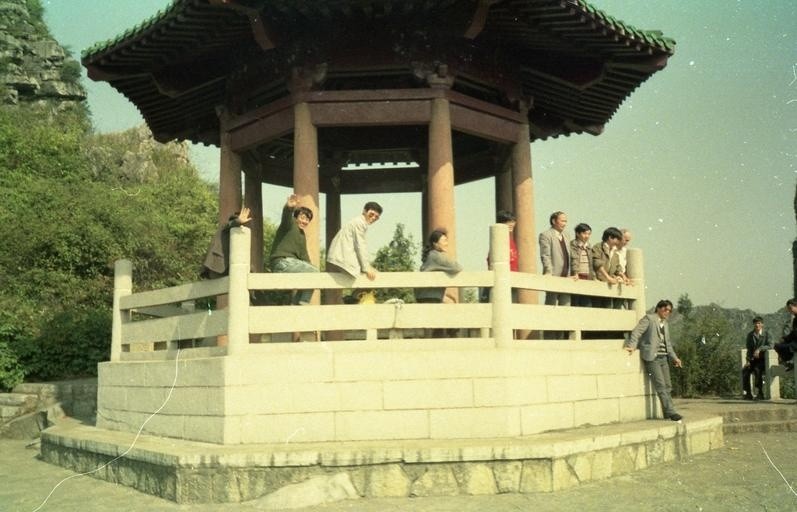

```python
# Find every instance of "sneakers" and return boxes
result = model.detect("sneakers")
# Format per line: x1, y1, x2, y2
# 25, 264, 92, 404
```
742, 394, 764, 400
664, 413, 682, 420
785, 363, 794, 372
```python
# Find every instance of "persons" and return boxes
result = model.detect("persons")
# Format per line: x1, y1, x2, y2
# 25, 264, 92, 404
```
773, 297, 797, 373
202, 205, 265, 306
537, 210, 573, 306
616, 227, 635, 310
569, 221, 595, 307
590, 226, 632, 307
326, 200, 383, 303
622, 298, 685, 422
412, 225, 463, 338
480, 209, 518, 303
269, 192, 321, 307
741, 316, 775, 402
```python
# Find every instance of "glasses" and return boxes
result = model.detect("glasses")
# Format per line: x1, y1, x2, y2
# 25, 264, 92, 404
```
662, 305, 672, 314
368, 211, 379, 219
